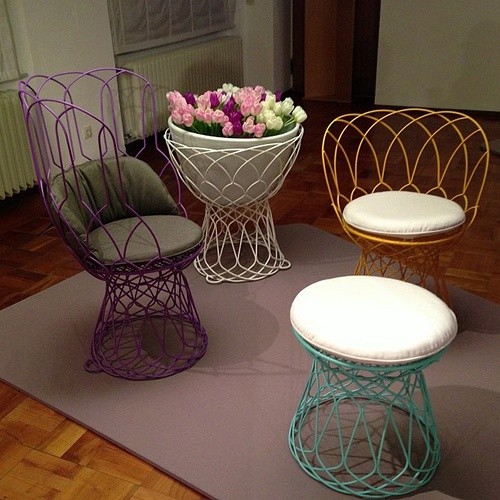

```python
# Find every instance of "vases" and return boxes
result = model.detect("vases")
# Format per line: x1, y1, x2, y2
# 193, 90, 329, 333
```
168, 115, 300, 206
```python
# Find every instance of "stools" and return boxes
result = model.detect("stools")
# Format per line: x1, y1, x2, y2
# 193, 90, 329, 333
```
289, 275, 458, 500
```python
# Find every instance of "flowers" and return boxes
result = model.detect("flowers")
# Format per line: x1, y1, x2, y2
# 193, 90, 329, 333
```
165, 82, 308, 138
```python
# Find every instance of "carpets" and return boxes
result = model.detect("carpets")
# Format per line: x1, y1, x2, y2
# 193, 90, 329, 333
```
0, 222, 500, 500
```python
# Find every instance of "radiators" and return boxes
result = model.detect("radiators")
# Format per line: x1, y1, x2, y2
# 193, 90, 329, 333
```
0, 88, 49, 200
118, 35, 243, 140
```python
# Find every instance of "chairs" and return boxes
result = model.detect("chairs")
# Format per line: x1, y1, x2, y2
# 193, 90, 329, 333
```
320, 108, 491, 306
17, 67, 209, 383
